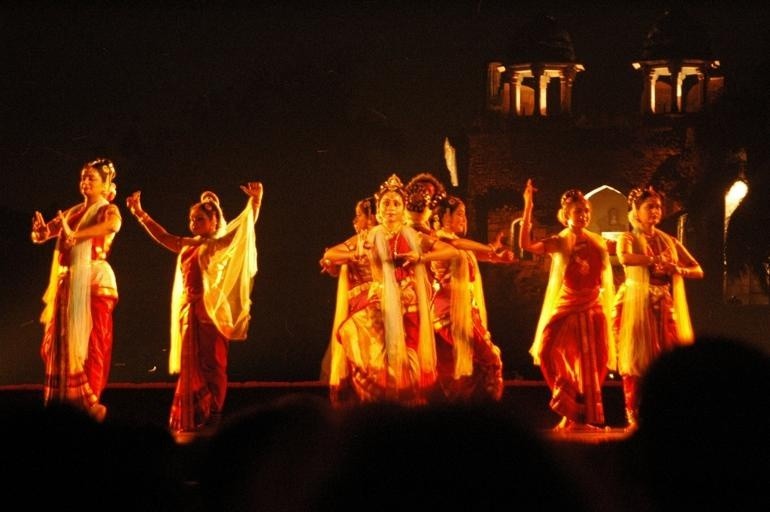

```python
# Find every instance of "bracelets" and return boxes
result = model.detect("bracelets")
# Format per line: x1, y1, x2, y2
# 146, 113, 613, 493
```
648, 256, 655, 266
682, 267, 689, 279
138, 212, 149, 223
251, 201, 261, 208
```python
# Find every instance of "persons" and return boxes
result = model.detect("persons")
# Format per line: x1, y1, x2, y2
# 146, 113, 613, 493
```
0, 335, 770, 511
27, 155, 125, 425
126, 180, 264, 444
613, 181, 705, 434
517, 175, 618, 435
315, 169, 517, 416
